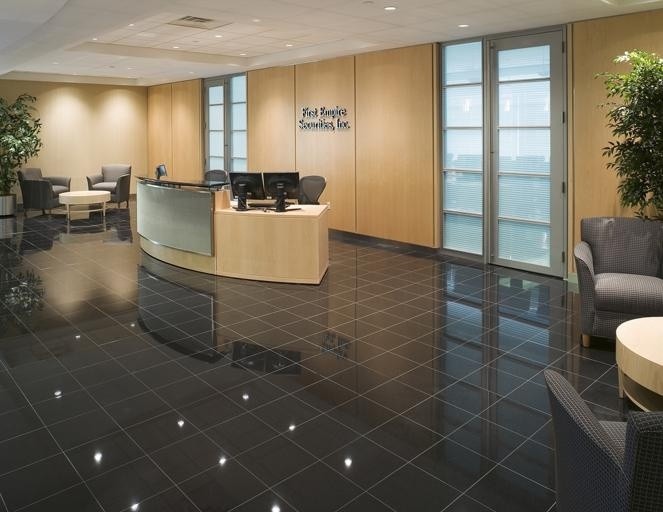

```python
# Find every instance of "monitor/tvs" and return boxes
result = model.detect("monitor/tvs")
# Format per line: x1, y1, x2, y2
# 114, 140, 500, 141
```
263, 172, 300, 211
156, 165, 167, 180
229, 172, 264, 211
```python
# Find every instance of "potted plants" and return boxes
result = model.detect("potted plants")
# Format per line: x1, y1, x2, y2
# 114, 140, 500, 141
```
0, 93, 44, 216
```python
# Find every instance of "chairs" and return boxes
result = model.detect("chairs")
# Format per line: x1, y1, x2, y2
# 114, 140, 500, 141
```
204, 169, 227, 191
296, 175, 327, 204
86, 163, 131, 212
572, 216, 663, 348
17, 167, 72, 217
543, 366, 663, 512
